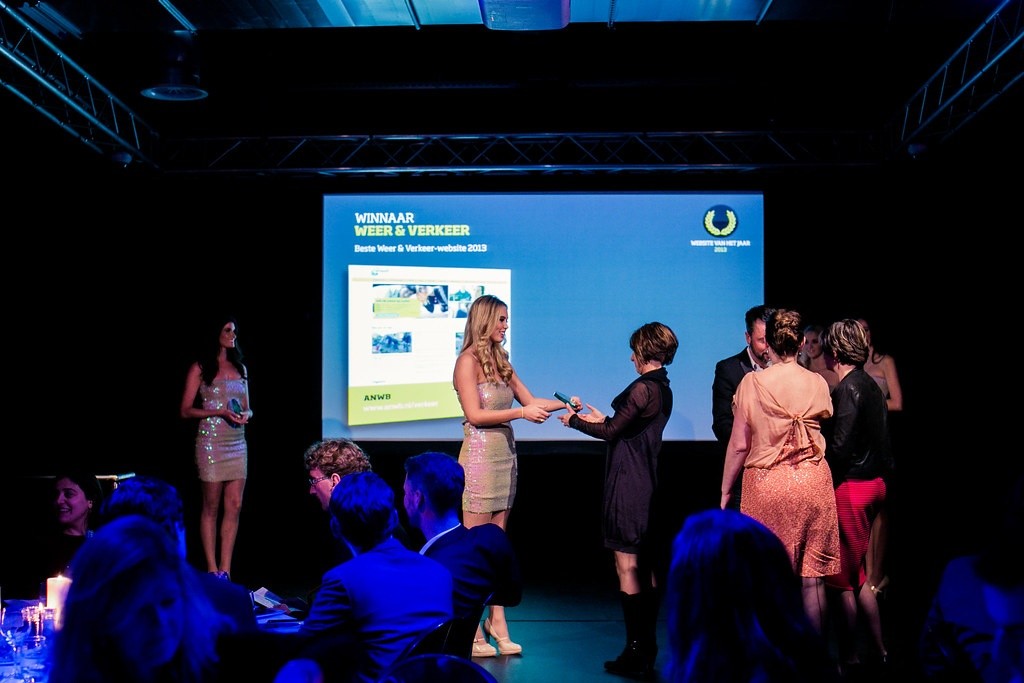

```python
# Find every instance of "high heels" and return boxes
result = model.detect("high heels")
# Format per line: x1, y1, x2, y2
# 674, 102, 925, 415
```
483, 618, 522, 656
470, 624, 497, 657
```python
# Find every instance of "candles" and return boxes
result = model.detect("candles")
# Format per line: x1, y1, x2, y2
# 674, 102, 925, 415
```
47, 574, 73, 608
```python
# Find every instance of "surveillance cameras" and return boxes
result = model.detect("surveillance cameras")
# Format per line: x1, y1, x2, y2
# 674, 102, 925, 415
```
112, 153, 132, 167
908, 144, 927, 159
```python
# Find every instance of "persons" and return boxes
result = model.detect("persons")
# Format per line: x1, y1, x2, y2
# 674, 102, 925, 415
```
712, 305, 902, 675
179, 312, 253, 582
271, 437, 511, 683
558, 322, 679, 674
28, 469, 271, 683
453, 295, 584, 660
660, 508, 841, 683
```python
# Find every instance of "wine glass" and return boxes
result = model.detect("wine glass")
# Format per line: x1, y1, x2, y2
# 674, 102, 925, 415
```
0, 607, 54, 683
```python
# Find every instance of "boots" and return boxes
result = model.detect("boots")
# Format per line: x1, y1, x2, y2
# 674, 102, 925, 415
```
604, 590, 648, 673
619, 590, 662, 682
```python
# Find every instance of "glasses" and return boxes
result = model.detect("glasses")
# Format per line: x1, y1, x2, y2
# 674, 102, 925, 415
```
307, 473, 344, 486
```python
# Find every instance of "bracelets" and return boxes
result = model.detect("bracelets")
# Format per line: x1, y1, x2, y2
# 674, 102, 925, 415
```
521, 406, 523, 418
